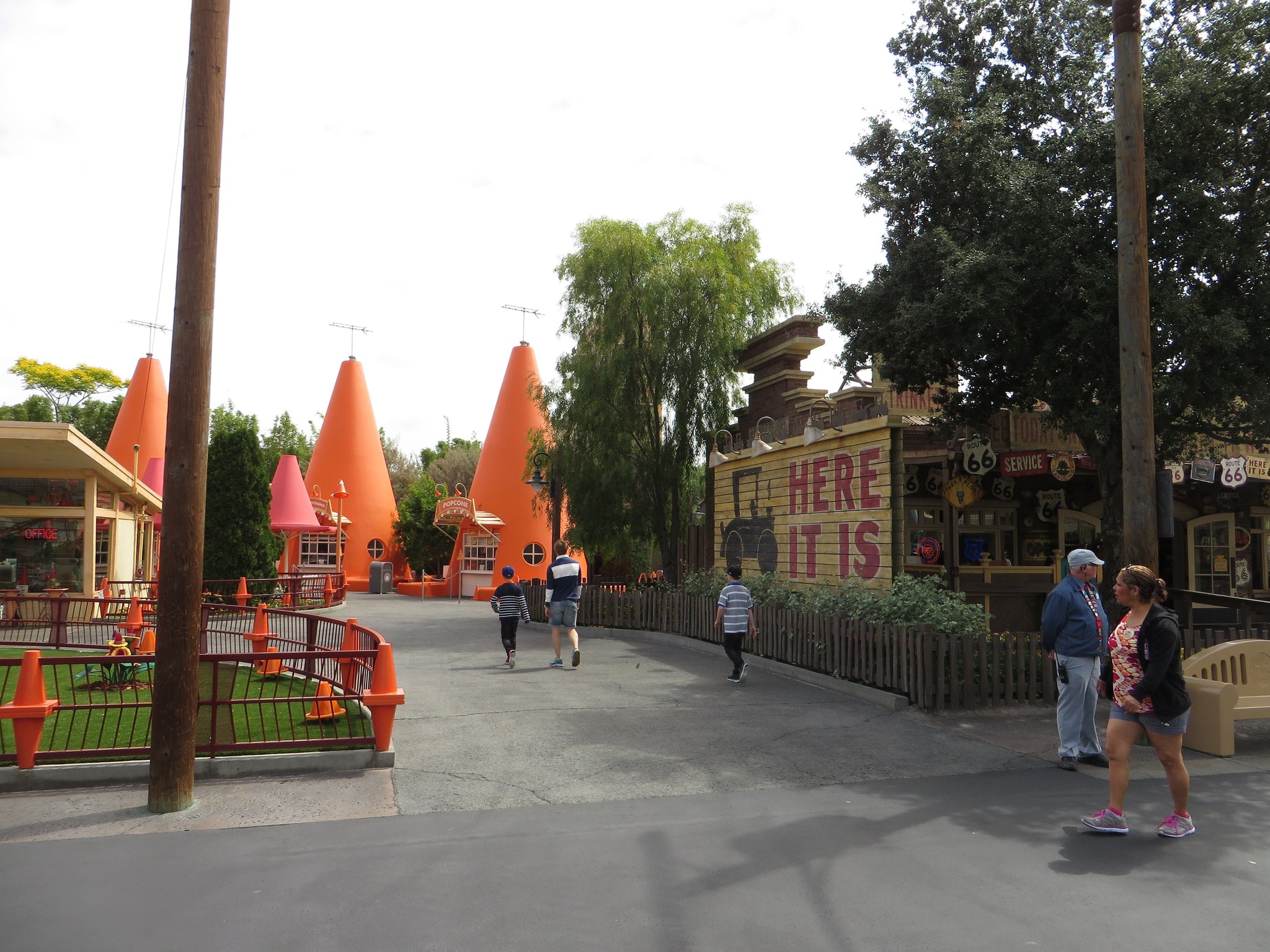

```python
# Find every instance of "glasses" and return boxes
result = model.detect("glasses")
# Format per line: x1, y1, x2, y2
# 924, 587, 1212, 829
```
1086, 564, 1098, 568
1126, 564, 1146, 589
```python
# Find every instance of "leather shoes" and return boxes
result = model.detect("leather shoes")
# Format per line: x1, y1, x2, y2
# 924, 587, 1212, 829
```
1061, 756, 1078, 771
1077, 754, 1109, 768
1081, 809, 1129, 834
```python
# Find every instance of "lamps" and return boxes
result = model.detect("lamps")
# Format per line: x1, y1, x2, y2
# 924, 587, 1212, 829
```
751, 416, 786, 459
803, 399, 843, 448
709, 429, 741, 469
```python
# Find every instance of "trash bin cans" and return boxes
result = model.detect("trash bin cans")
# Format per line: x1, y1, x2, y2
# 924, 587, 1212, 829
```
368, 561, 394, 594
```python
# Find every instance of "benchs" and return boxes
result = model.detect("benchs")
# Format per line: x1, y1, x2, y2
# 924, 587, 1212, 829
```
1181, 639, 1270, 757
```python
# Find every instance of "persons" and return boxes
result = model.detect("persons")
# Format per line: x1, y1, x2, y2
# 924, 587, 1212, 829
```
1001, 551, 1012, 567
714, 565, 758, 683
1040, 547, 1115, 772
544, 540, 582, 667
490, 566, 531, 668
1081, 565, 1195, 837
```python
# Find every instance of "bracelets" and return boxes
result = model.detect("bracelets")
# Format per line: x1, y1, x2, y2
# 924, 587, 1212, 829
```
544, 607, 549, 609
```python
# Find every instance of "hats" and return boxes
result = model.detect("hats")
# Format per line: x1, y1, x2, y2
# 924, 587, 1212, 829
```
501, 565, 514, 577
723, 565, 742, 578
1067, 549, 1105, 567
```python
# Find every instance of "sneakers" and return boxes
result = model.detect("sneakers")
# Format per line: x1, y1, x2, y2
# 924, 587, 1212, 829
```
505, 649, 516, 668
549, 657, 564, 667
571, 648, 581, 667
1157, 812, 1196, 837
739, 661, 751, 683
727, 669, 740, 682
